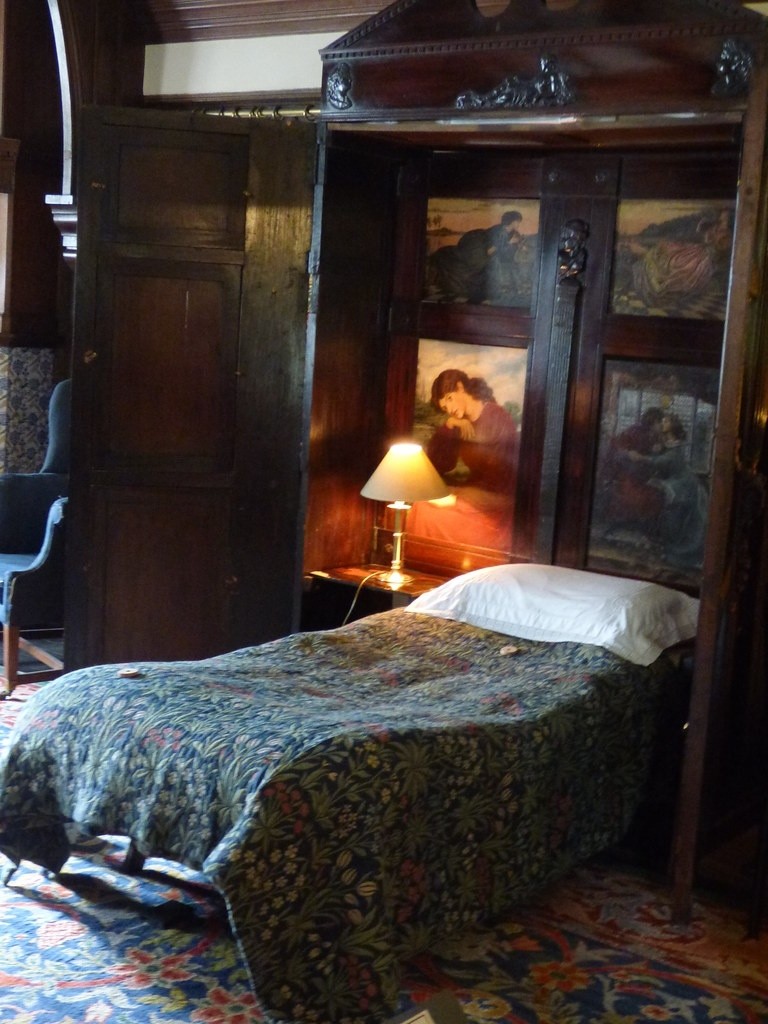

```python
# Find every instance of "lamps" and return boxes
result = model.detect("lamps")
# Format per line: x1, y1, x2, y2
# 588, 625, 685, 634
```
359, 437, 447, 584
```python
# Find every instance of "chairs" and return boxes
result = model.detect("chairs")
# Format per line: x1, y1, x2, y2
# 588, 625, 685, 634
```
0, 606, 700, 1024
1, 378, 70, 698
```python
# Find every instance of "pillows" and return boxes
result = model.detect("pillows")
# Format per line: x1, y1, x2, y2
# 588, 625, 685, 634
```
403, 562, 700, 667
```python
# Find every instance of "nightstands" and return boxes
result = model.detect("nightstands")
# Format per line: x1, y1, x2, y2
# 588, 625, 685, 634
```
309, 563, 452, 633
